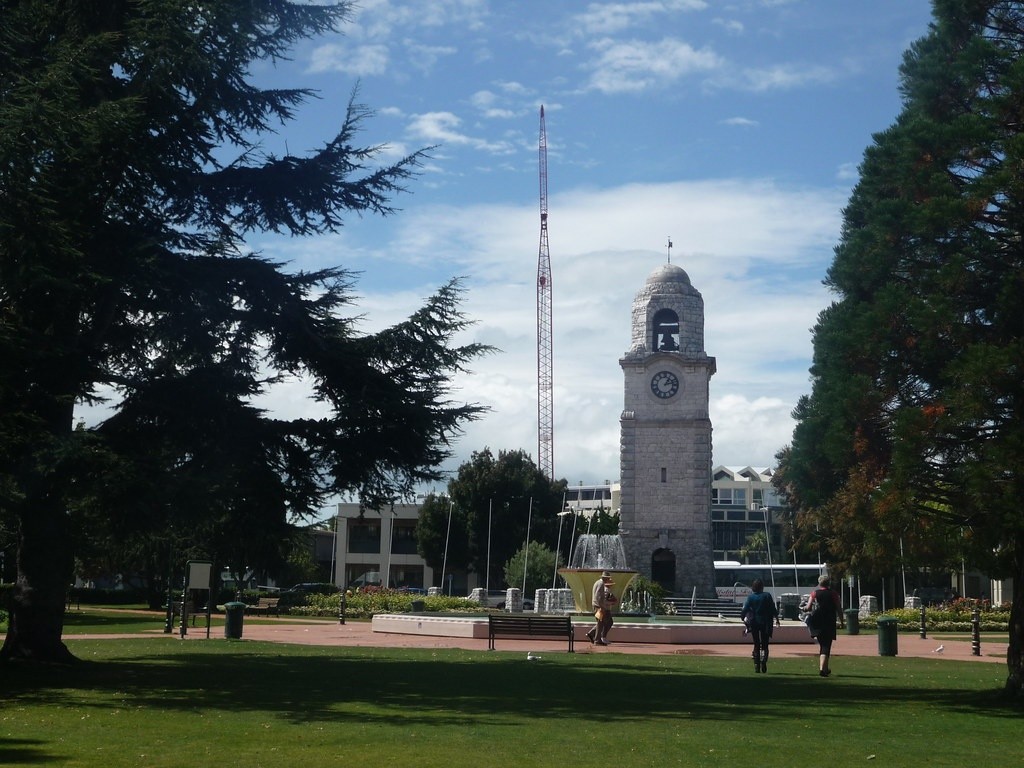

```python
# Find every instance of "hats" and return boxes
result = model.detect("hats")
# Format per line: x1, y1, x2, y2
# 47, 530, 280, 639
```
604, 578, 616, 586
599, 572, 612, 578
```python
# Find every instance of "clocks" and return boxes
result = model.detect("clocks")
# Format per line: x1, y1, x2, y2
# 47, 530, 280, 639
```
645, 365, 686, 405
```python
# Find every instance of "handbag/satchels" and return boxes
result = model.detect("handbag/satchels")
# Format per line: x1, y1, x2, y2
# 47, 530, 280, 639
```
595, 608, 607, 622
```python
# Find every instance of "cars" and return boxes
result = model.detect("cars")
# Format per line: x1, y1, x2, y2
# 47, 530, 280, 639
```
460, 590, 506, 610
523, 599, 535, 609
406, 589, 427, 595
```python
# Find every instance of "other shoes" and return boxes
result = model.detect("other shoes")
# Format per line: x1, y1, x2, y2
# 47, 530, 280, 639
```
586, 633, 594, 644
601, 637, 611, 644
761, 661, 767, 673
755, 663, 761, 673
596, 640, 607, 646
819, 669, 831, 677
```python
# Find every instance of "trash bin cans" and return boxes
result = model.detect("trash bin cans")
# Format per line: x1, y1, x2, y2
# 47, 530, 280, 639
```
411, 600, 425, 612
223, 602, 246, 639
844, 608, 860, 634
875, 616, 900, 656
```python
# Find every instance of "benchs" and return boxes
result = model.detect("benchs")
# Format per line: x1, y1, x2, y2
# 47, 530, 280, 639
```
172, 602, 197, 627
487, 613, 575, 653
248, 597, 280, 618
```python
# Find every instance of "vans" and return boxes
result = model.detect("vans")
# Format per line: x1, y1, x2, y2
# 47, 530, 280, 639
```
913, 588, 960, 606
288, 584, 330, 593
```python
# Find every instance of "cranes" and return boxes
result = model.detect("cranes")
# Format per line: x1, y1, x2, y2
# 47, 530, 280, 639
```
535, 103, 555, 482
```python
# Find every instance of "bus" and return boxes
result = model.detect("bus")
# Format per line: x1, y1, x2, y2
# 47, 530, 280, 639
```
713, 560, 830, 610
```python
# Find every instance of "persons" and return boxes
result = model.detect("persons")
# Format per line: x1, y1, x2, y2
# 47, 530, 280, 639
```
585, 570, 616, 646
739, 577, 781, 673
942, 587, 960, 602
803, 574, 844, 676
402, 584, 410, 593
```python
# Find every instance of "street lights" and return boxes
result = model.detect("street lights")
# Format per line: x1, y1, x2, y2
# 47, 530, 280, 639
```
312, 504, 336, 532
441, 500, 455, 592
760, 505, 778, 610
